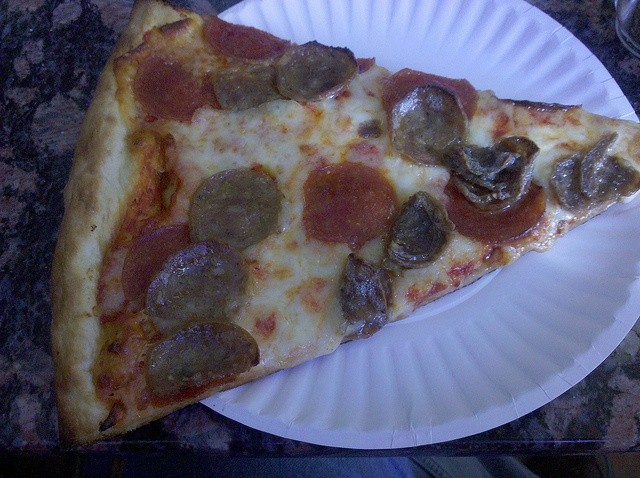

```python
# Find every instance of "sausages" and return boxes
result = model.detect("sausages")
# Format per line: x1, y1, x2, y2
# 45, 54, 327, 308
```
122, 224, 181, 307
204, 13, 291, 58
382, 67, 478, 131
503, 98, 583, 114
446, 173, 547, 239
359, 56, 374, 70
134, 51, 216, 122
306, 165, 396, 243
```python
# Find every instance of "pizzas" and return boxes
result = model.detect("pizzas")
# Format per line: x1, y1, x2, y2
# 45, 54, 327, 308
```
53, 1, 639, 446
